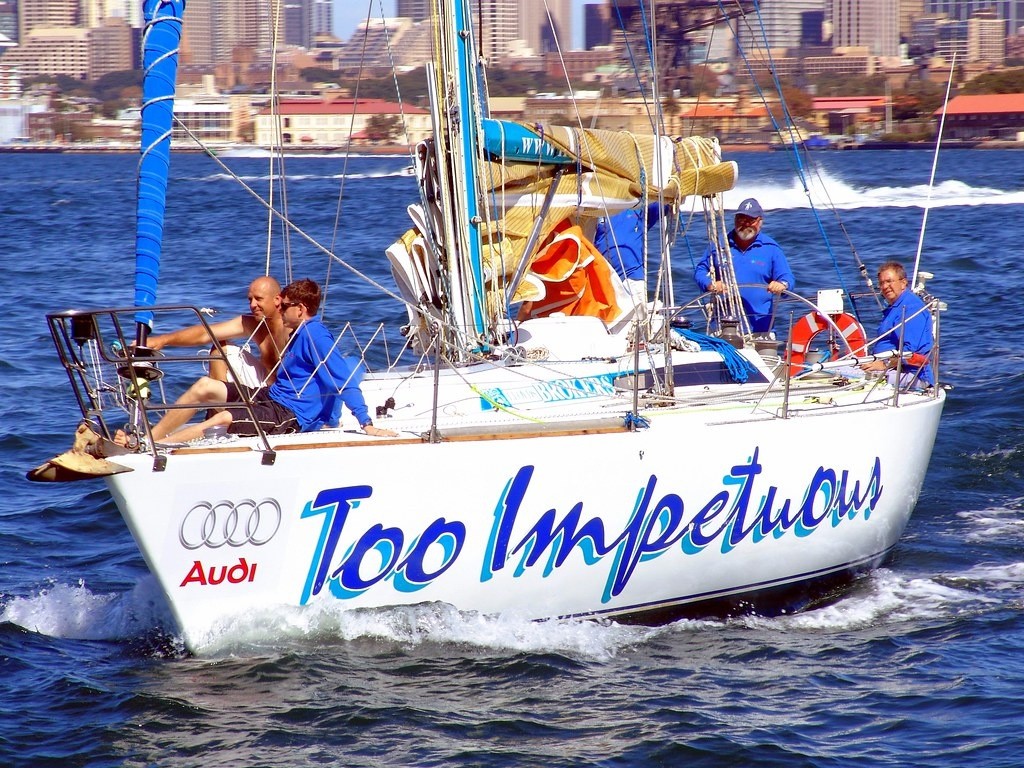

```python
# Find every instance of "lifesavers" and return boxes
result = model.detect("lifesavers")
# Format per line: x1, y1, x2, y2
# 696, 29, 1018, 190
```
784, 310, 867, 377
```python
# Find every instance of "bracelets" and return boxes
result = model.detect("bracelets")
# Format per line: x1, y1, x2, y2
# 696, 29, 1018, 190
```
361, 422, 373, 429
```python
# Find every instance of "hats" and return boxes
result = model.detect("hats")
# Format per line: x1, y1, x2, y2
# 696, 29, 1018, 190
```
734, 198, 765, 219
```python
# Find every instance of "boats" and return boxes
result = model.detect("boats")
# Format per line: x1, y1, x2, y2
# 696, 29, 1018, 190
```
40, 0, 966, 668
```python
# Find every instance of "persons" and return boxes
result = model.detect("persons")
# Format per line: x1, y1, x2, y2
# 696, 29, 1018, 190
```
114, 278, 400, 449
693, 198, 796, 338
859, 261, 935, 392
131, 275, 293, 422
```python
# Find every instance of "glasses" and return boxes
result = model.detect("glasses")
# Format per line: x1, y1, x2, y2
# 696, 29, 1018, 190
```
735, 215, 761, 223
280, 301, 310, 312
876, 276, 906, 288
